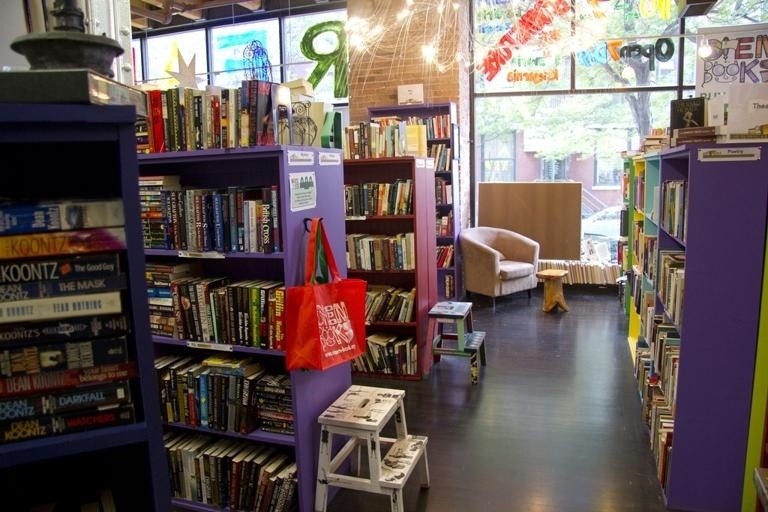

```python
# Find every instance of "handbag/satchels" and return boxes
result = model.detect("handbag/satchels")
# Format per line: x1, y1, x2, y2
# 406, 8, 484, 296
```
282, 215, 366, 373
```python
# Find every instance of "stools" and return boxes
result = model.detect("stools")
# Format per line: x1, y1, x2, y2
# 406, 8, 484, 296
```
536, 269, 570, 313
423, 301, 487, 385
314, 386, 430, 512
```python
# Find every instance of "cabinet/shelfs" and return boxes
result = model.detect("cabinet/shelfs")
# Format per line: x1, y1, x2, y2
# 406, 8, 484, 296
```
137, 141, 351, 511
1, 101, 174, 511
424, 115, 461, 303
614, 124, 766, 512
341, 153, 436, 382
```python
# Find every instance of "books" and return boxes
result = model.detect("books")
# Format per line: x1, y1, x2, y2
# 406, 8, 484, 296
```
535, 258, 619, 287
437, 244, 454, 299
0, 195, 138, 447
139, 176, 282, 252
436, 210, 453, 237
154, 352, 294, 435
347, 232, 415, 270
616, 167, 691, 501
619, 123, 768, 160
163, 428, 300, 512
668, 96, 710, 150
344, 179, 413, 216
352, 332, 418, 375
365, 285, 416, 323
146, 260, 287, 351
137, 79, 333, 153
427, 143, 451, 171
343, 122, 427, 159
434, 177, 452, 204
371, 114, 450, 139
751, 464, 767, 512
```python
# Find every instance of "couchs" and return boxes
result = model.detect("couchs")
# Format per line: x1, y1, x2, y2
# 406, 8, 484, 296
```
460, 226, 540, 314
